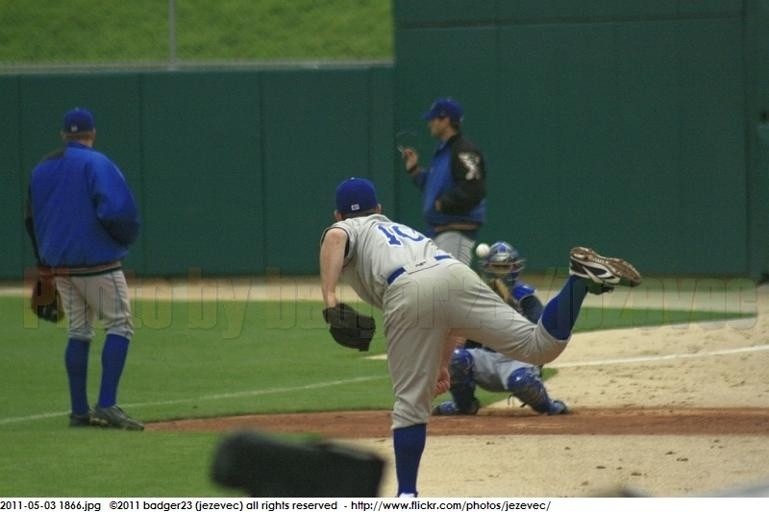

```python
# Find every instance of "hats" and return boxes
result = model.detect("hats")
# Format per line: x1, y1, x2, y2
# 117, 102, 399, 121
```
336, 178, 377, 213
63, 109, 94, 132
423, 99, 463, 122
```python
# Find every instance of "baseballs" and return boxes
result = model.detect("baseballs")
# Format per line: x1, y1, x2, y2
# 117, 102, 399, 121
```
477, 243, 489, 258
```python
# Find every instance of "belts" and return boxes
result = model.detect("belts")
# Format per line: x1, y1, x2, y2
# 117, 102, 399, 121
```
388, 255, 451, 285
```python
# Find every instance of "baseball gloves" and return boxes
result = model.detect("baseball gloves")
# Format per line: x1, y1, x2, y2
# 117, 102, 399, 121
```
31, 283, 65, 322
324, 304, 374, 352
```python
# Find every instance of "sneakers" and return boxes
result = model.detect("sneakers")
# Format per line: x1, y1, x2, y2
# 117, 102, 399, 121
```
70, 408, 103, 427
90, 404, 144, 430
432, 399, 479, 414
546, 400, 568, 416
569, 247, 641, 286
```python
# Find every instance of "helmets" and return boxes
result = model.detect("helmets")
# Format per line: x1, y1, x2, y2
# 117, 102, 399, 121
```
478, 242, 525, 286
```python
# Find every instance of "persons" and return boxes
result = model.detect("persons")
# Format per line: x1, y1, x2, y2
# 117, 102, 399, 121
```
401, 95, 487, 269
22, 106, 146, 432
431, 240, 567, 417
319, 176, 643, 498
